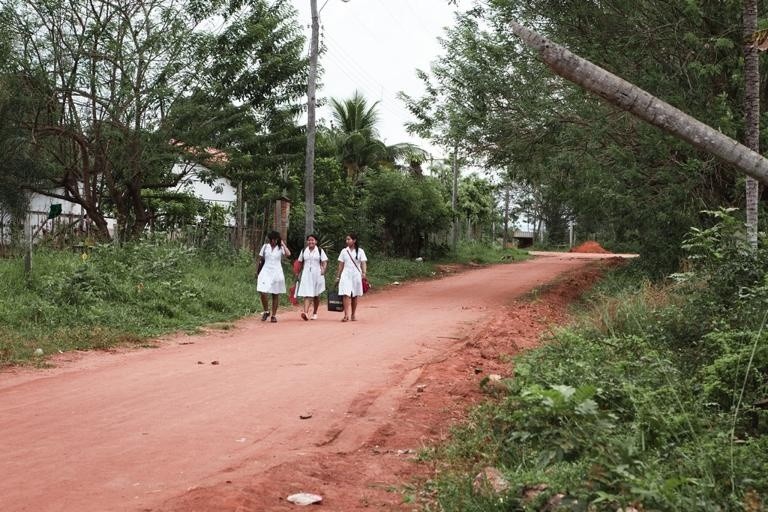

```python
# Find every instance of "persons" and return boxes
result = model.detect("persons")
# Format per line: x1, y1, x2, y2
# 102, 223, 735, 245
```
334, 233, 368, 322
295, 235, 329, 321
255, 231, 291, 322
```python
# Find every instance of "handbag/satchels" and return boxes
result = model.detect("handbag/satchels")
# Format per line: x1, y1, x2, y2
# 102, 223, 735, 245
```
328, 293, 344, 312
289, 286, 298, 304
256, 256, 266, 276
361, 278, 369, 293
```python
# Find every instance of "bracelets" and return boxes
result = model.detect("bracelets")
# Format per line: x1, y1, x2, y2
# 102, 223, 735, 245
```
336, 271, 340, 280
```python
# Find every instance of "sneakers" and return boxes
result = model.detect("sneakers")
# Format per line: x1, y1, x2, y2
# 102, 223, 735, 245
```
260, 310, 277, 323
300, 312, 317, 322
341, 314, 359, 322
